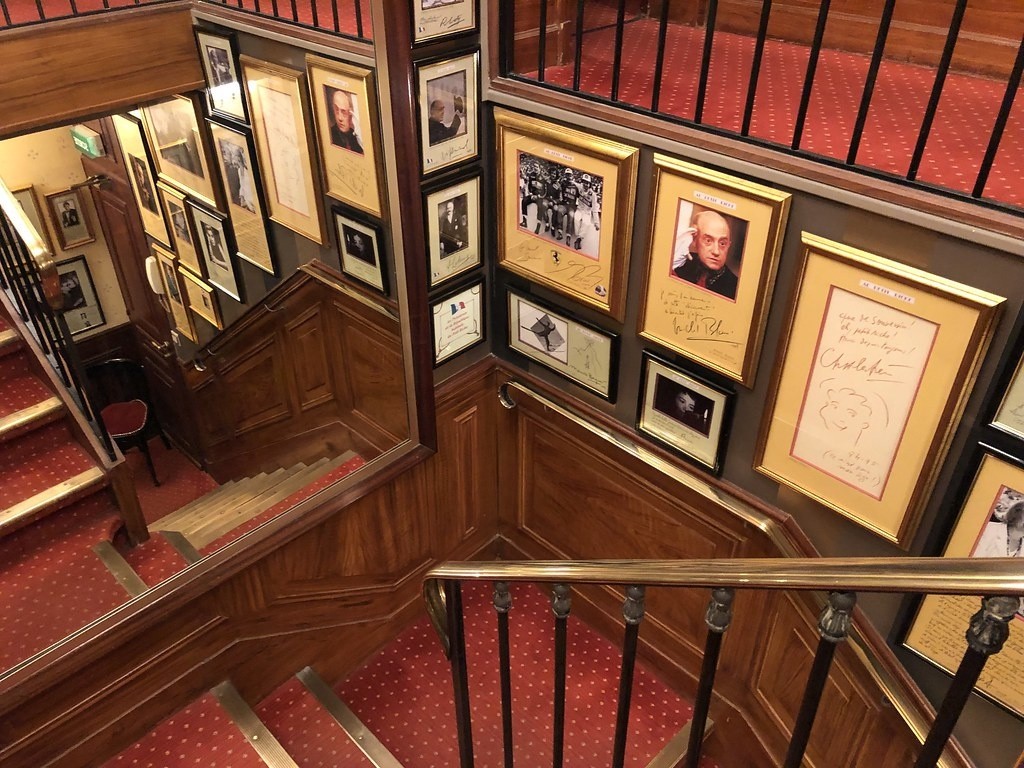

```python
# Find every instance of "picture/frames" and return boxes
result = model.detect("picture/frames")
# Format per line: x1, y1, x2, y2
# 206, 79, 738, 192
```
185, 199, 243, 303
635, 348, 736, 479
330, 204, 389, 296
191, 24, 249, 125
139, 91, 226, 215
177, 265, 224, 331
893, 304, 1024, 724
151, 243, 200, 343
156, 180, 208, 282
205, 116, 280, 276
10, 254, 106, 341
750, 230, 1010, 553
412, 42, 483, 182
304, 53, 385, 220
420, 167, 484, 292
237, 51, 329, 247
428, 273, 487, 370
41, 188, 97, 251
0, 186, 56, 269
409, 0, 480, 49
491, 105, 639, 325
635, 152, 793, 391
111, 113, 176, 253
506, 281, 620, 404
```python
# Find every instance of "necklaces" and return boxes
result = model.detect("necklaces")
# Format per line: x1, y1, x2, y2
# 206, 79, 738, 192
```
1006, 522, 1021, 557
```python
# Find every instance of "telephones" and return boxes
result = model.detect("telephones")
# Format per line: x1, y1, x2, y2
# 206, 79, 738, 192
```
145, 256, 165, 295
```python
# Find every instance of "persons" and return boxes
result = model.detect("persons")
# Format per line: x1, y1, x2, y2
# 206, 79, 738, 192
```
208, 48, 227, 86
329, 90, 364, 154
428, 95, 464, 144
61, 201, 80, 227
665, 388, 696, 423
61, 277, 87, 311
346, 233, 373, 263
224, 146, 255, 214
438, 201, 464, 252
203, 225, 227, 269
973, 501, 1023, 559
672, 211, 738, 299
519, 157, 600, 251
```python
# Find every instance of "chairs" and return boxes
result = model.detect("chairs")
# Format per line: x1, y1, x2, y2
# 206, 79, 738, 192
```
84, 358, 172, 486
